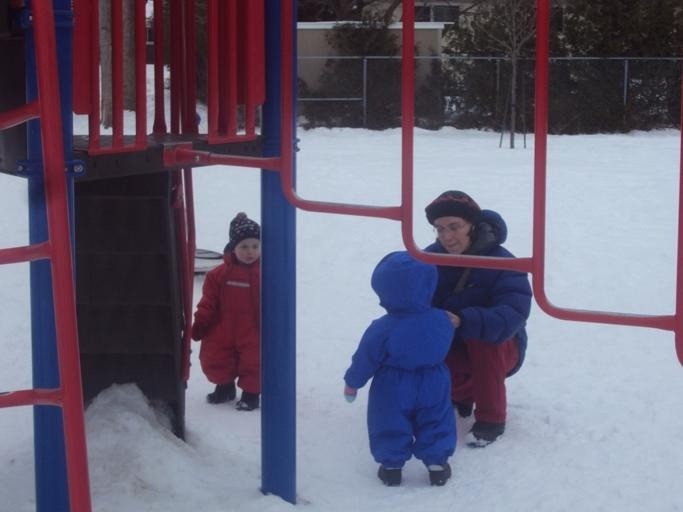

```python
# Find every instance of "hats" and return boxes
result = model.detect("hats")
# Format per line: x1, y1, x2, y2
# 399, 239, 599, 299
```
227, 212, 260, 251
425, 190, 483, 225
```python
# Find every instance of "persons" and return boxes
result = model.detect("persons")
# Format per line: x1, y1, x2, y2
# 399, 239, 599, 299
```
340, 250, 456, 488
192, 210, 260, 412
422, 189, 532, 449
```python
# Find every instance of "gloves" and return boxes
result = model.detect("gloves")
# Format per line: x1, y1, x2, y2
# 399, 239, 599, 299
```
191, 318, 210, 342
344, 382, 357, 403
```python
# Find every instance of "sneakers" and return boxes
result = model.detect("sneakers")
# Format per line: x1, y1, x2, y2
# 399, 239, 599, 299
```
464, 420, 506, 446
378, 463, 402, 487
429, 462, 451, 487
206, 382, 236, 404
455, 403, 474, 416
234, 391, 260, 412
